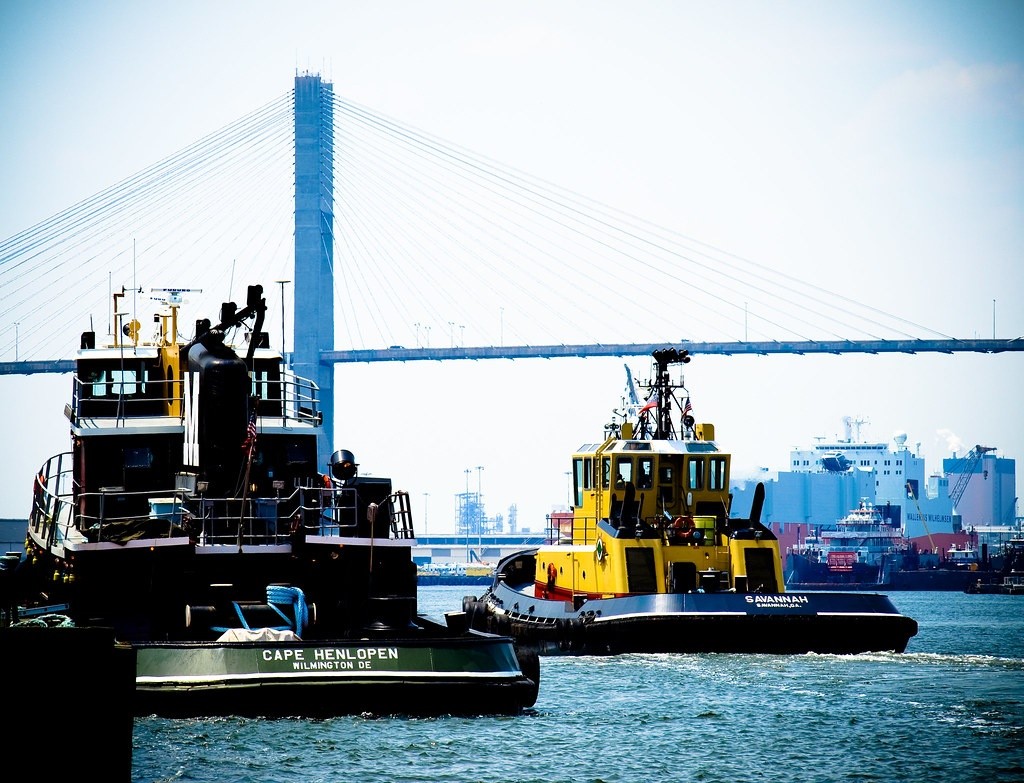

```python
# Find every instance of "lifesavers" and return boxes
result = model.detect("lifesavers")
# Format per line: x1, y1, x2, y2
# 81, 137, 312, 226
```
547, 562, 556, 583
674, 515, 695, 538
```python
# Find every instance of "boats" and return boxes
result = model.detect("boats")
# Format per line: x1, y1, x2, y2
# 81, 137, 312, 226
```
1, 281, 543, 721
783, 495, 1024, 594
459, 343, 919, 655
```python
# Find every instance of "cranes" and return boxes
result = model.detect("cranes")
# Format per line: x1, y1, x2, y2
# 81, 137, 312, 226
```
943, 445, 997, 508
